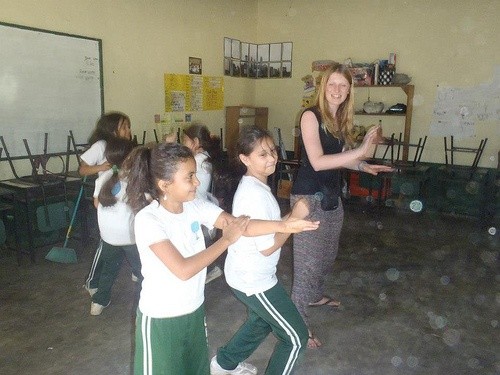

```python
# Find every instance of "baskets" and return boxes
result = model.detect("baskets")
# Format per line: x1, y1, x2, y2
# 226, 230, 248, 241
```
346, 67, 375, 86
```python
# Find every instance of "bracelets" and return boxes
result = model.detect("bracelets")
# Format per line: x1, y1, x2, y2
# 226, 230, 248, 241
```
358, 161, 363, 170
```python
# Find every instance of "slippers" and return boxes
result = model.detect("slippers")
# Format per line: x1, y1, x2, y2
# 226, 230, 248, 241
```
306, 334, 322, 349
308, 296, 340, 306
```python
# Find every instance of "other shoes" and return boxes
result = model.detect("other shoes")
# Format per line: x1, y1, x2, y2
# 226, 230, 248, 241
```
131, 272, 139, 282
90, 300, 111, 315
85, 285, 99, 299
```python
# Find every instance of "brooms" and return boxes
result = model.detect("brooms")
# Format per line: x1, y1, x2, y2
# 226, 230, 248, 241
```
44, 175, 88, 265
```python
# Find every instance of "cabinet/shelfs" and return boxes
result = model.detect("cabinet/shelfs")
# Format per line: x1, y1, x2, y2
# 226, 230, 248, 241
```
352, 83, 415, 162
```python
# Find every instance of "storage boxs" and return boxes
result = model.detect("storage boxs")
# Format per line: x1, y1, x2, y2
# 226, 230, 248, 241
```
348, 63, 397, 85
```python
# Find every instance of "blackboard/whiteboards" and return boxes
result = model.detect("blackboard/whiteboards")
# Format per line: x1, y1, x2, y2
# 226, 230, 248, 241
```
0, 22, 105, 161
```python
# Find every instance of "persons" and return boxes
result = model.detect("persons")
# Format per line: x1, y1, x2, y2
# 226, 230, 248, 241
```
290, 63, 392, 350
127, 142, 320, 375
165, 124, 223, 284
207, 128, 309, 375
89, 137, 155, 315
79, 112, 138, 295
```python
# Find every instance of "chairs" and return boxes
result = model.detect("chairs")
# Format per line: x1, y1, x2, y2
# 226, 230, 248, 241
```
275, 128, 500, 177
0, 128, 222, 226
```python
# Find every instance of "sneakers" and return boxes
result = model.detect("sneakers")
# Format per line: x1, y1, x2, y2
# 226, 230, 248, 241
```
210, 354, 258, 375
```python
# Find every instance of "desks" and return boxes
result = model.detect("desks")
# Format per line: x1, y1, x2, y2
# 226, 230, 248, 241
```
270, 166, 499, 225
0, 171, 108, 265
